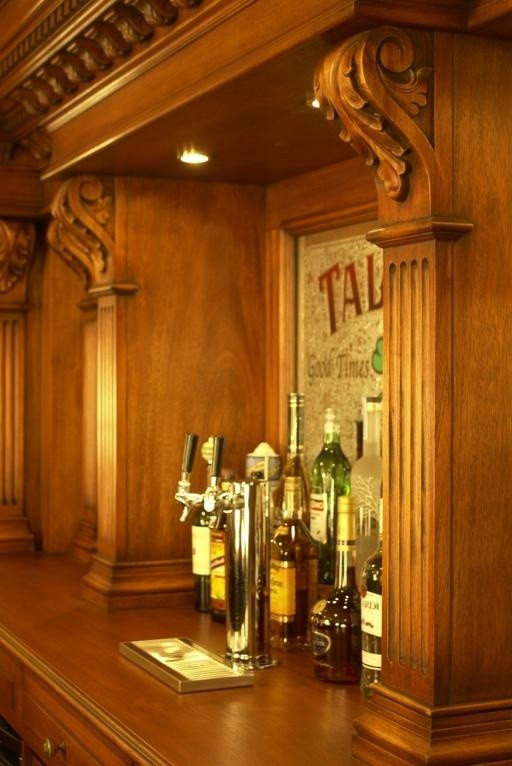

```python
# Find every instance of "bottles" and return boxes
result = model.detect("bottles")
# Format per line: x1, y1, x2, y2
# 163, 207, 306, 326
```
191, 390, 383, 698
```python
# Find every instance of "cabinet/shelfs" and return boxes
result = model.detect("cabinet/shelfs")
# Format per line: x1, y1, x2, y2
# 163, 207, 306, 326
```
0, 638, 141, 766
